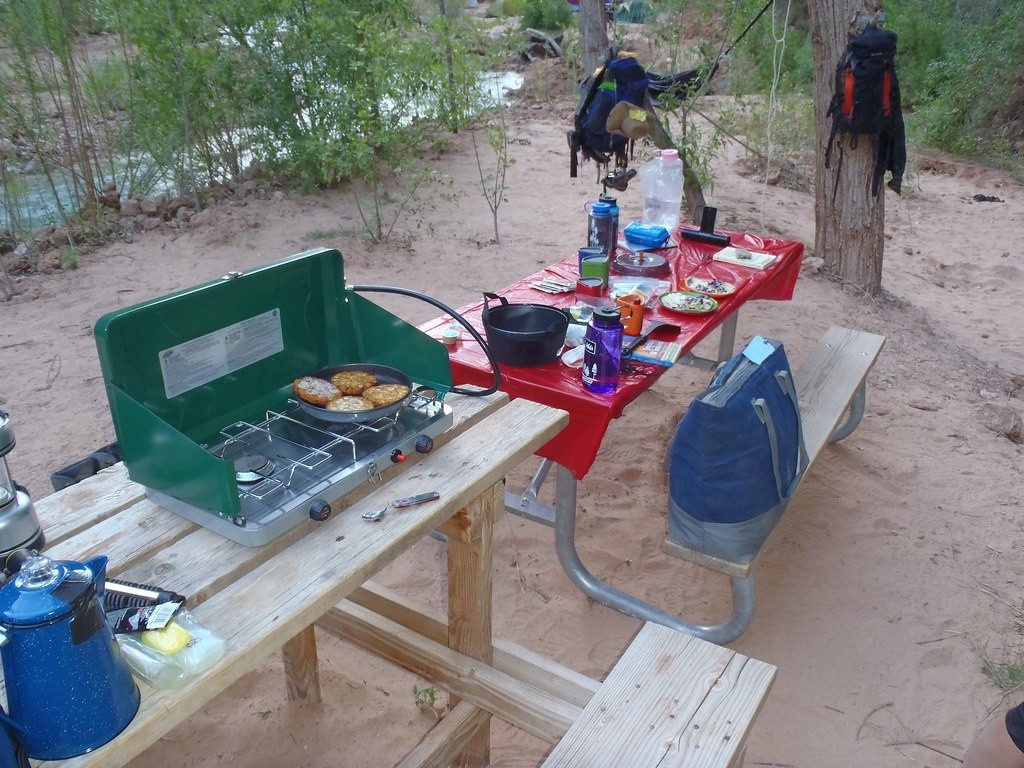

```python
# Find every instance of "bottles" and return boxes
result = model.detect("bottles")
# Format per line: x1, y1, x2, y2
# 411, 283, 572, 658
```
581, 305, 624, 394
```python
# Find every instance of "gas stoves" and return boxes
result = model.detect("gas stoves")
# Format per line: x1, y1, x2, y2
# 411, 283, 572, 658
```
93, 246, 455, 549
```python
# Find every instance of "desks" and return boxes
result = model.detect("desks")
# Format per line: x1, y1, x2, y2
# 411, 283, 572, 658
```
0, 383, 570, 768
415, 219, 805, 611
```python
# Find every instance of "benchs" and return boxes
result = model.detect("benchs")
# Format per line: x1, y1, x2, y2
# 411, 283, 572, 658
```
642, 325, 887, 645
540, 620, 777, 768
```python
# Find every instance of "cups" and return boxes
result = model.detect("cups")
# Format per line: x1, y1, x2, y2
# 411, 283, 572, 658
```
581, 254, 610, 290
648, 148, 684, 233
575, 277, 604, 305
599, 192, 619, 251
584, 199, 614, 255
578, 247, 603, 276
616, 294, 645, 336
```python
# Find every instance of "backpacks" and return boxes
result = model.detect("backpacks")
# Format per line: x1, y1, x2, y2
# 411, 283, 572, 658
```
570, 57, 650, 164
825, 21, 898, 136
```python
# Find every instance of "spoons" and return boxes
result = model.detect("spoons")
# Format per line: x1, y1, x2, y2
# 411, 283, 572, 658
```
361, 491, 442, 520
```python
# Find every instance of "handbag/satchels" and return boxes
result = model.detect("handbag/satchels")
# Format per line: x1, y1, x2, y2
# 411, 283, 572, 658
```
663, 336, 809, 565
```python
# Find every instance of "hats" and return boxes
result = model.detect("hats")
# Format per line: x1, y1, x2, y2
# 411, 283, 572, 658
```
605, 100, 656, 140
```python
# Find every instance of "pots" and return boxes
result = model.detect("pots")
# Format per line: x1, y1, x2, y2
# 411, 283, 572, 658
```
481, 291, 571, 366
295, 362, 413, 423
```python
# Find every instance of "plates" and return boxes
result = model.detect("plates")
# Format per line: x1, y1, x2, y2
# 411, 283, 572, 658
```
658, 291, 718, 313
685, 276, 736, 298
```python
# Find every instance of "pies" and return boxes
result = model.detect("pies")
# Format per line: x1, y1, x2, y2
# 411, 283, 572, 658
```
293, 370, 410, 411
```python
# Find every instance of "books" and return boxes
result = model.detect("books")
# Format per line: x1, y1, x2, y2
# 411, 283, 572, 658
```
621, 336, 681, 366
712, 246, 777, 270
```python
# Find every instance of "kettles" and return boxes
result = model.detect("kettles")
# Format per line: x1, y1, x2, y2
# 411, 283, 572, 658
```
0, 552, 141, 761
